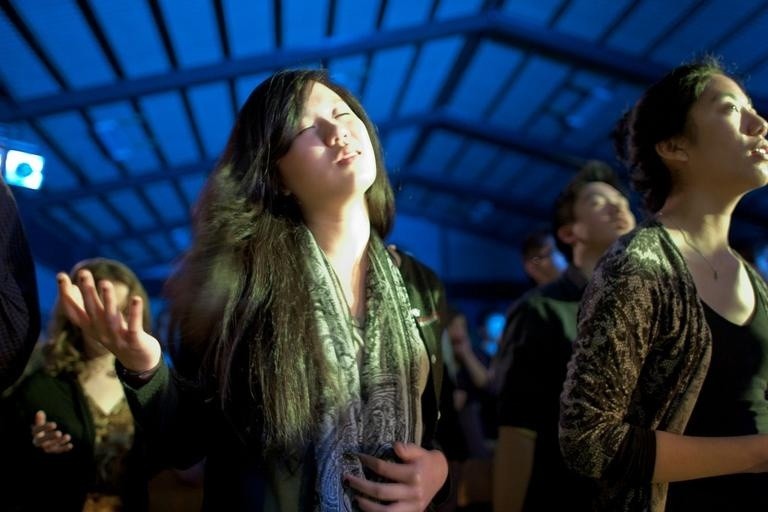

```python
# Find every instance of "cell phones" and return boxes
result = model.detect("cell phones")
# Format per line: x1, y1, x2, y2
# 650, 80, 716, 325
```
0, 138, 48, 199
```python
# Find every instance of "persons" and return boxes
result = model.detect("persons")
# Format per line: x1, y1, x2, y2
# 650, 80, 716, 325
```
56, 64, 458, 511
1, 173, 153, 512
447, 45, 768, 512
